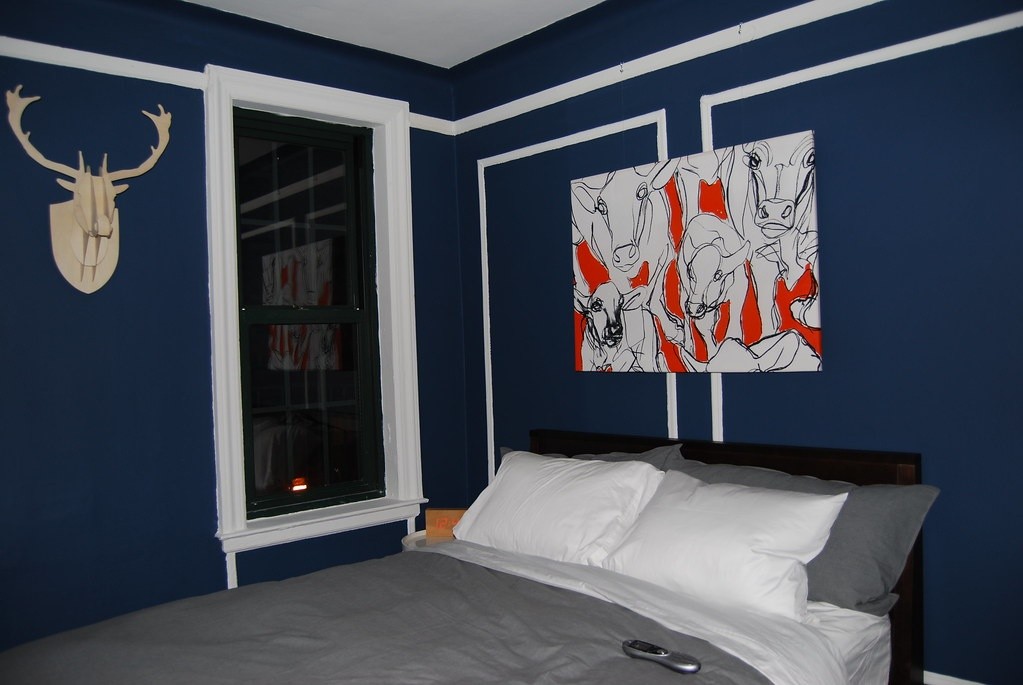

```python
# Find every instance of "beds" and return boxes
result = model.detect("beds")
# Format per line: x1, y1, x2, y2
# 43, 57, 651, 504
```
0, 421, 927, 684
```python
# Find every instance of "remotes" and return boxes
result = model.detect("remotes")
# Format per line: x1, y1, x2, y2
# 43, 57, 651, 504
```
622, 639, 701, 674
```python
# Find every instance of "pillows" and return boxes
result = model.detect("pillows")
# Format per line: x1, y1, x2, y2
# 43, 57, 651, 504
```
662, 458, 942, 623
598, 468, 851, 635
498, 442, 683, 473
455, 450, 666, 572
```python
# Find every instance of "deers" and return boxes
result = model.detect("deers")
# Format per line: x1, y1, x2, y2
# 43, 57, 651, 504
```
3, 82, 173, 295
